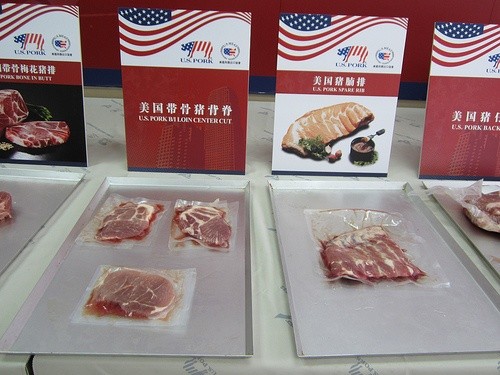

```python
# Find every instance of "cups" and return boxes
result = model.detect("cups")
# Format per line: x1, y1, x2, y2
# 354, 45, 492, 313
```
350, 137, 375, 165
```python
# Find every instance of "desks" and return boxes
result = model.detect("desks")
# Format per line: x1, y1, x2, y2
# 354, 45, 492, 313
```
0, 96, 500, 375
